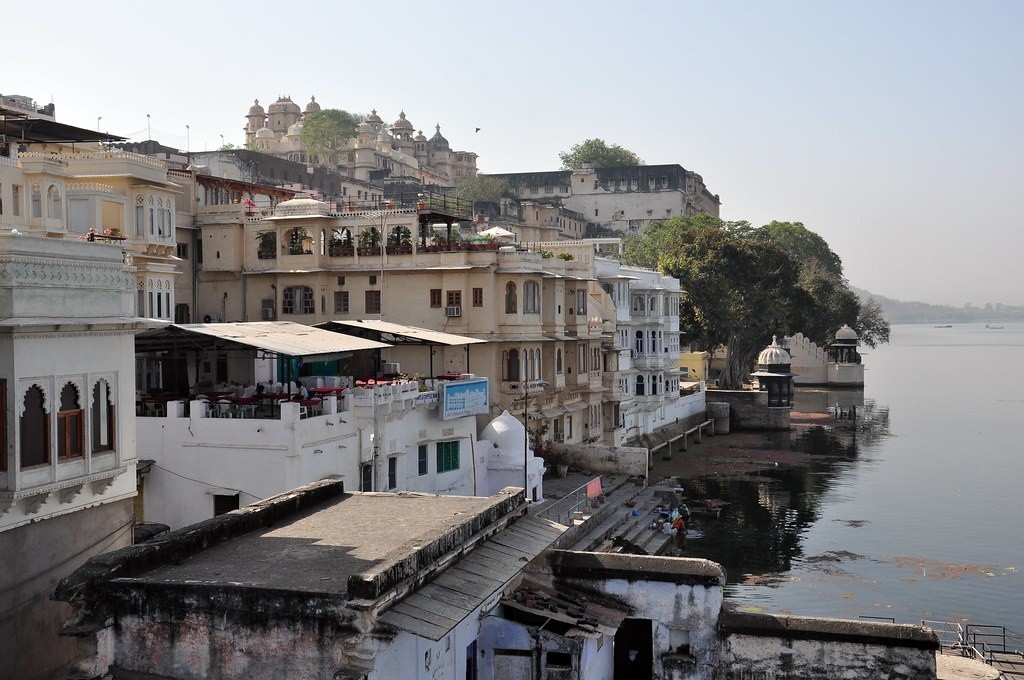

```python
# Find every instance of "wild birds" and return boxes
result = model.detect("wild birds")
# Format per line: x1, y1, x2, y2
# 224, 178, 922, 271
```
476, 127, 482, 133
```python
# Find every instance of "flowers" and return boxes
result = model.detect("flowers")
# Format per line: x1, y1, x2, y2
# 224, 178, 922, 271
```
418, 193, 424, 202
78, 226, 129, 242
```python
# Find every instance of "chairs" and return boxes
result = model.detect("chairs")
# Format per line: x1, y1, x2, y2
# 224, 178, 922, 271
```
310, 397, 323, 417
196, 395, 208, 398
143, 400, 165, 417
300, 406, 308, 419
339, 387, 349, 412
367, 379, 375, 388
195, 398, 216, 418
217, 400, 240, 418
237, 385, 256, 418
218, 381, 298, 394
355, 380, 366, 388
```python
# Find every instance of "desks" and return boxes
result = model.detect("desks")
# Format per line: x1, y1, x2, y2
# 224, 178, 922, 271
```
140, 387, 345, 417
353, 375, 464, 388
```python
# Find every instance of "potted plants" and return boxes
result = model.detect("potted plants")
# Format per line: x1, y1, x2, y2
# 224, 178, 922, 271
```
255, 225, 500, 259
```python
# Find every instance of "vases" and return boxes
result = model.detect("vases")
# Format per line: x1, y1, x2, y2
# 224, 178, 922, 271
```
386, 203, 393, 209
348, 206, 356, 211
556, 464, 568, 478
417, 202, 425, 209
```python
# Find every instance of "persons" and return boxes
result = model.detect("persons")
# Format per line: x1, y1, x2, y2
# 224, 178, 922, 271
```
222, 364, 227, 382
242, 379, 275, 396
672, 514, 690, 537
229, 381, 235, 386
679, 503, 690, 518
292, 381, 308, 400
706, 498, 724, 512
223, 384, 228, 388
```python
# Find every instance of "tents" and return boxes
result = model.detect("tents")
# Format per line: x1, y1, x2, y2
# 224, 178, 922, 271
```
309, 319, 488, 389
135, 321, 394, 401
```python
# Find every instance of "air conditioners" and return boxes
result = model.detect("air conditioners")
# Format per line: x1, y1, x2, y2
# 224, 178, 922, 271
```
444, 306, 460, 317
262, 308, 272, 319
200, 313, 220, 323
555, 432, 564, 441
